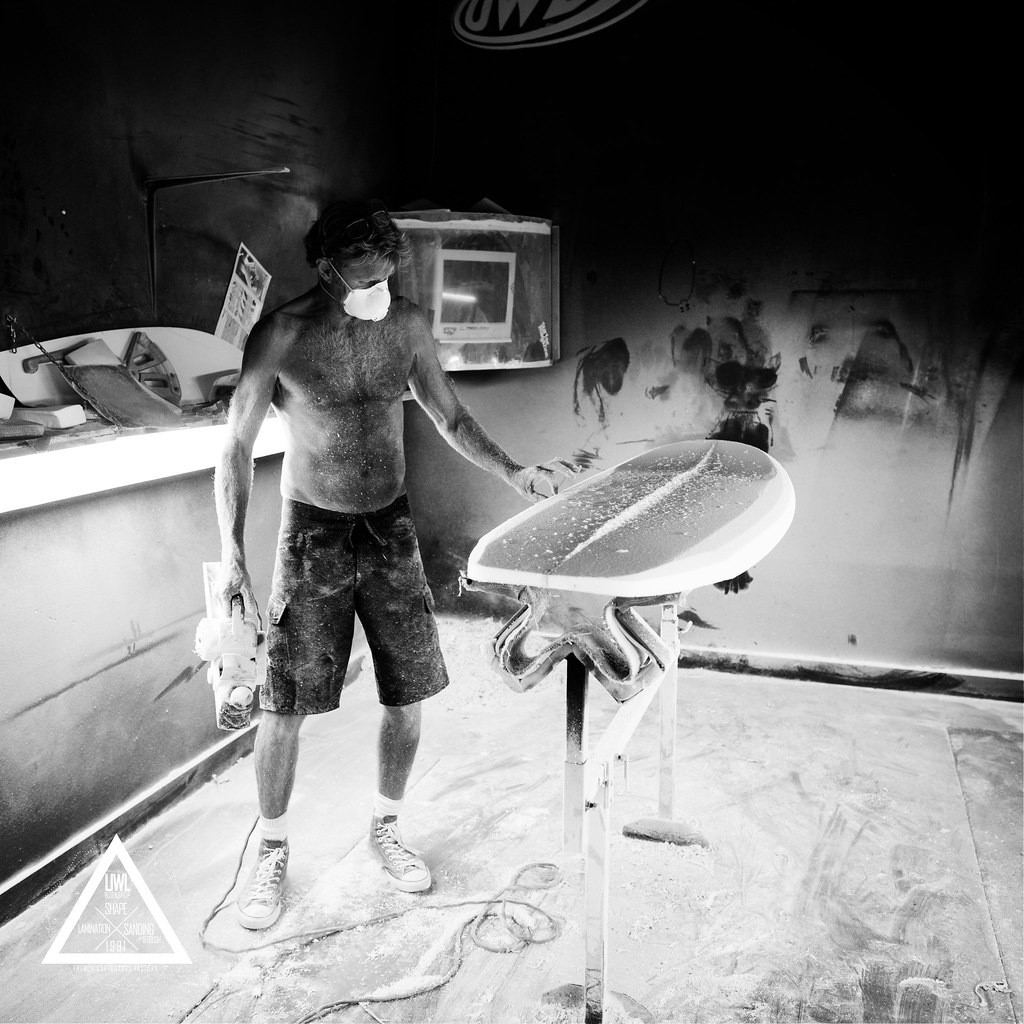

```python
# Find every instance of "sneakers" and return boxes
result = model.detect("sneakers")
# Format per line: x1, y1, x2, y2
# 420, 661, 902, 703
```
235, 838, 290, 930
369, 813, 431, 893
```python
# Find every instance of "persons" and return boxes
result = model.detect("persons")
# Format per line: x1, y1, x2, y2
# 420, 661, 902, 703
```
214, 199, 581, 930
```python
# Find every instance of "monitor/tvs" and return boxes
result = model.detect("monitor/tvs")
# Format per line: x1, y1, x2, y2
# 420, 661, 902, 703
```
430, 248, 516, 345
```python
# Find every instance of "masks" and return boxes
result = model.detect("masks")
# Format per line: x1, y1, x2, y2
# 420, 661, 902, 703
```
315, 259, 392, 322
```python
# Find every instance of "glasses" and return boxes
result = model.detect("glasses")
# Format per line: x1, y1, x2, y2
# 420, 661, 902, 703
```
337, 211, 390, 242
